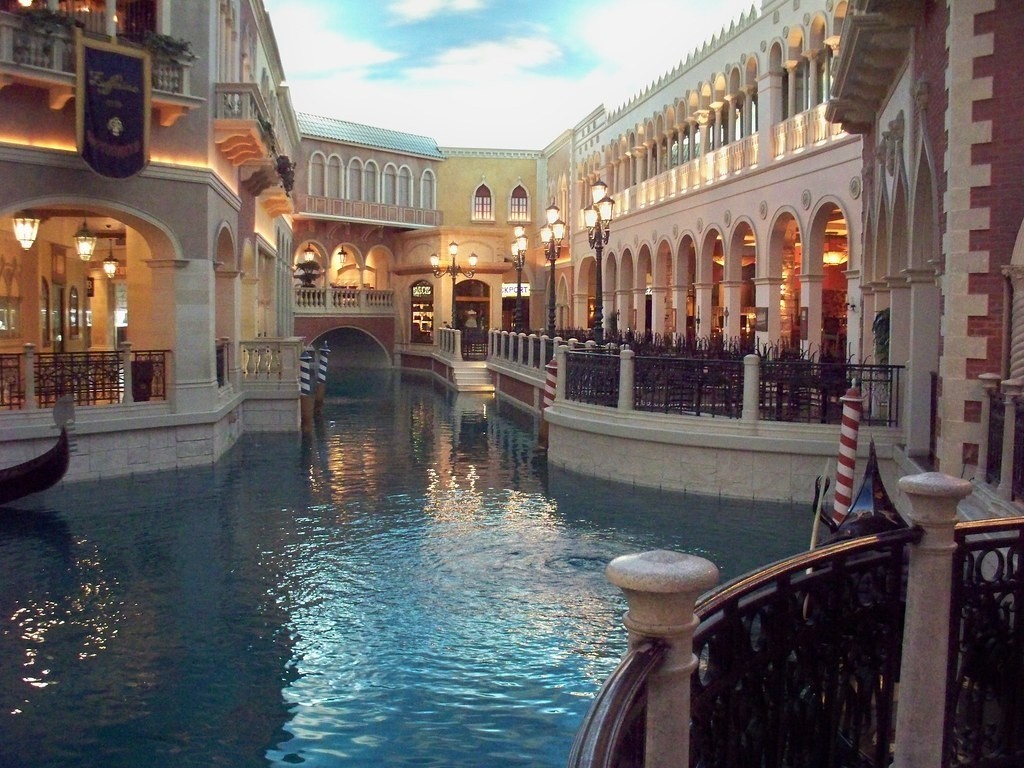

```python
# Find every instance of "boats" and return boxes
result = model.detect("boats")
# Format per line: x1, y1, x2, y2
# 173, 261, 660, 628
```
814, 436, 910, 548
0, 425, 71, 505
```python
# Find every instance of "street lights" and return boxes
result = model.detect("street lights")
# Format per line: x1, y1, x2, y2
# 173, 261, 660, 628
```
511, 220, 528, 333
539, 198, 566, 339
583, 176, 617, 347
430, 240, 479, 330
